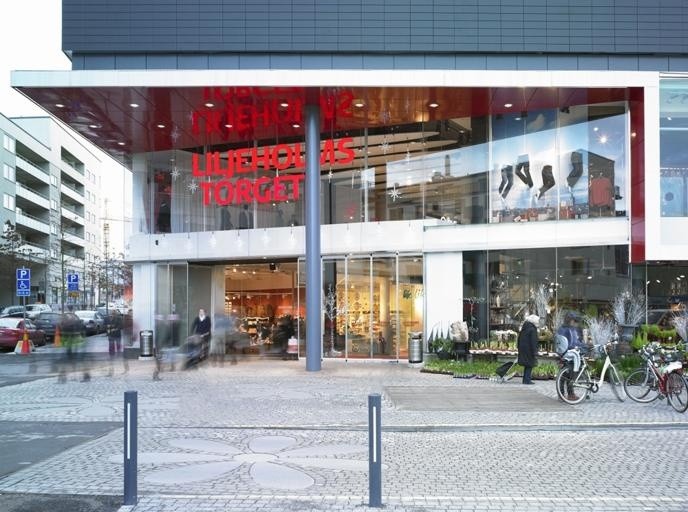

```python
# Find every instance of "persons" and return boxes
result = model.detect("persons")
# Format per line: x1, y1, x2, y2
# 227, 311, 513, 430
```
157, 202, 170, 231
566, 152, 583, 192
499, 165, 514, 202
427, 205, 441, 219
220, 205, 232, 230
276, 210, 298, 227
190, 306, 211, 357
106, 308, 121, 358
557, 312, 594, 400
535, 166, 555, 204
239, 204, 253, 229
518, 314, 540, 384
515, 154, 533, 192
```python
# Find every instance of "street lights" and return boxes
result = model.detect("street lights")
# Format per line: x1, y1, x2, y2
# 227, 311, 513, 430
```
51, 257, 64, 313
29, 252, 44, 268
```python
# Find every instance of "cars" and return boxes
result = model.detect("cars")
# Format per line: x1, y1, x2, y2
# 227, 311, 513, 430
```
0, 300, 133, 350
632, 309, 688, 349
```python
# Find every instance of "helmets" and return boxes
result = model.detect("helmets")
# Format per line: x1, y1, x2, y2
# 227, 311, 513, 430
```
566, 312, 581, 320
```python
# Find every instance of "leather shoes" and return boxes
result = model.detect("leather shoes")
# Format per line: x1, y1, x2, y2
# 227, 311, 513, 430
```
523, 380, 535, 384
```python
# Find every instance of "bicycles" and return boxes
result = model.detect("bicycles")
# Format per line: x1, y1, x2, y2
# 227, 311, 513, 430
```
554, 333, 688, 413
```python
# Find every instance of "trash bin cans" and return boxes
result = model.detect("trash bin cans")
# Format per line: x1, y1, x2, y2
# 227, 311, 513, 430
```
140, 329, 153, 357
407, 331, 423, 363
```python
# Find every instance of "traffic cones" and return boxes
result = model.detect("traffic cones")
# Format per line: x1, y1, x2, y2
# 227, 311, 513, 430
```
21, 326, 30, 353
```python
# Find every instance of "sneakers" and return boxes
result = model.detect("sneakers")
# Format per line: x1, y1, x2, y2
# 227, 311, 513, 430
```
558, 395, 580, 401
